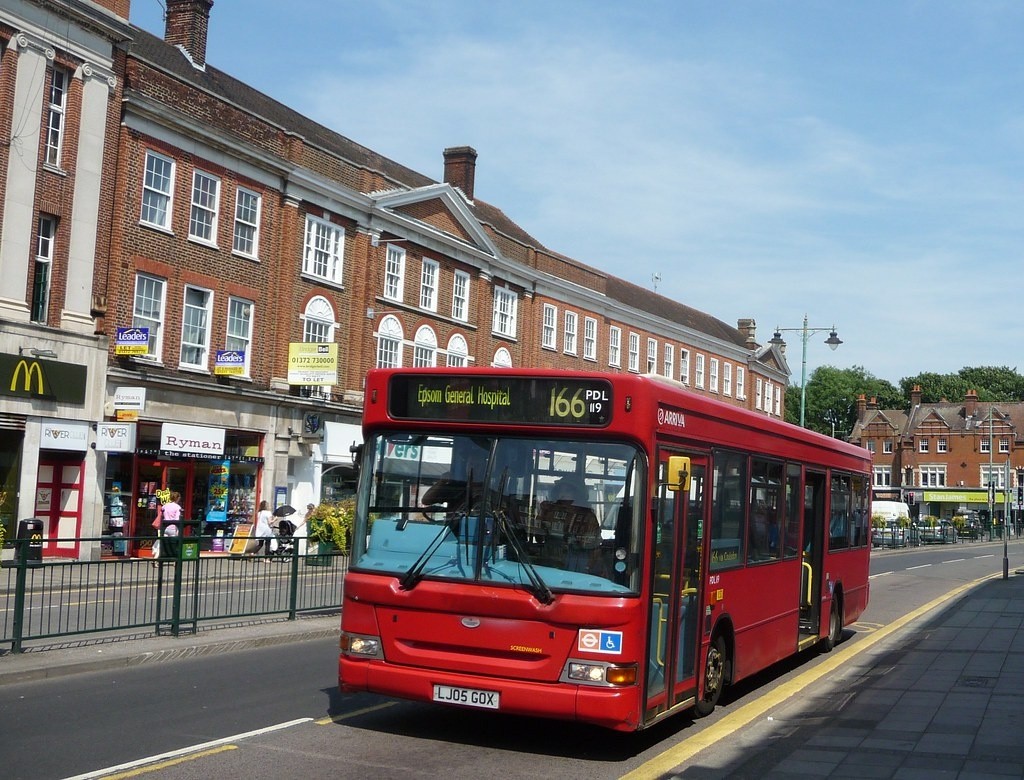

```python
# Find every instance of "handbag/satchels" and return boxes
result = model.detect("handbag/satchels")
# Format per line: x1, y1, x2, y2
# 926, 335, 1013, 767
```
152, 511, 162, 529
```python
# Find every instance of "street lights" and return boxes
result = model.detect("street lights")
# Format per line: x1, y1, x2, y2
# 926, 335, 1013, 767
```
970, 412, 1013, 546
771, 328, 842, 432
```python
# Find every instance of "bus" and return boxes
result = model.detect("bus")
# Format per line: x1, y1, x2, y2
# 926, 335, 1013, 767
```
336, 365, 873, 734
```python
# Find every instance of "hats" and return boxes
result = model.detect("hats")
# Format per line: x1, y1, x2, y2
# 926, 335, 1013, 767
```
556, 473, 586, 496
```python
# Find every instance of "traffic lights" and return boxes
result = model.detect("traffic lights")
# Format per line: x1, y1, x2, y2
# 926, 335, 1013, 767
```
908, 492, 915, 507
904, 494, 908, 503
987, 481, 995, 505
1018, 487, 1023, 505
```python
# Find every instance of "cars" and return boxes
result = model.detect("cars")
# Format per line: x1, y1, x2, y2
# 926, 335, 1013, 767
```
872, 519, 923, 548
916, 520, 959, 544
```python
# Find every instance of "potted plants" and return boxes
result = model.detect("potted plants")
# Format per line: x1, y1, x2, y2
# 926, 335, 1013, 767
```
307, 502, 352, 557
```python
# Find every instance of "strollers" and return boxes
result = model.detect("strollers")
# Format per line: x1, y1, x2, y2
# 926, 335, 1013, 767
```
271, 521, 299, 561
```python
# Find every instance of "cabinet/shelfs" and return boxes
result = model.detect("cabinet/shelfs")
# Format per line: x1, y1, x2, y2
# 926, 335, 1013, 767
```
227, 493, 254, 535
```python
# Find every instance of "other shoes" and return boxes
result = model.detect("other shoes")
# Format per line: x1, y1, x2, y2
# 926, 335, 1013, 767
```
263, 559, 272, 563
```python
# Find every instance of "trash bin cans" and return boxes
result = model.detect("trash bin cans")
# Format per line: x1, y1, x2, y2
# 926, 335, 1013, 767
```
14, 518, 43, 563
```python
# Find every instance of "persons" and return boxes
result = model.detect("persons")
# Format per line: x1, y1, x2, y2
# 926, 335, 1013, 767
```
250, 501, 277, 564
536, 476, 602, 577
421, 454, 506, 536
152, 492, 182, 568
275, 528, 289, 544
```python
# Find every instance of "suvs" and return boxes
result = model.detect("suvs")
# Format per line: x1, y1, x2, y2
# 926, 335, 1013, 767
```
953, 512, 983, 540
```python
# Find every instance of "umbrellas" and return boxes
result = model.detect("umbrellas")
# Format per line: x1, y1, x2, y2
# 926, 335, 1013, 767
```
273, 505, 296, 520
